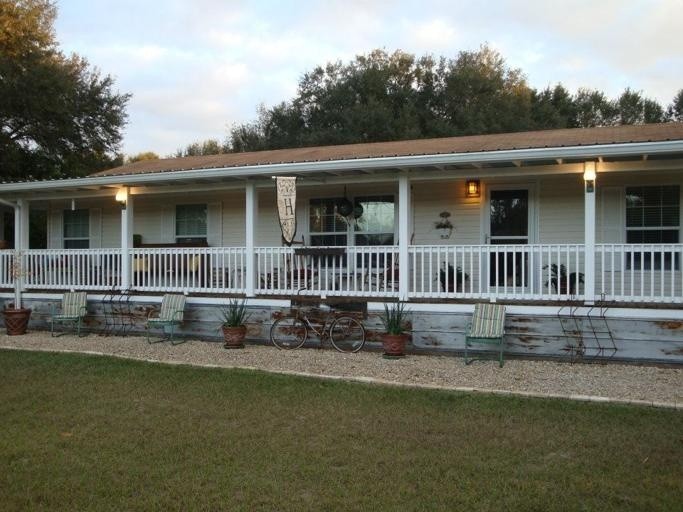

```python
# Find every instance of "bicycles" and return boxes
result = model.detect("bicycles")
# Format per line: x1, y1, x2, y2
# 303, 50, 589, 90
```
270, 286, 366, 355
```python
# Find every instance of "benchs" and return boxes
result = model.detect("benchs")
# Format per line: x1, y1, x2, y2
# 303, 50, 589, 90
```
130, 242, 209, 289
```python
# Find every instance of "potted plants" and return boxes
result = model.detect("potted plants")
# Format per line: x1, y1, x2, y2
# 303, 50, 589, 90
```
215, 293, 254, 349
375, 293, 416, 359
433, 261, 470, 292
434, 219, 453, 238
0, 248, 32, 336
542, 263, 585, 294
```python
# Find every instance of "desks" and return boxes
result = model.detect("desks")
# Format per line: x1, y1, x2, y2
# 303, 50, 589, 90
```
330, 272, 361, 291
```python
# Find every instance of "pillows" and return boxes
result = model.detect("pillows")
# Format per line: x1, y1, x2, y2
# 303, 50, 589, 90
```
133, 257, 150, 273
189, 254, 199, 273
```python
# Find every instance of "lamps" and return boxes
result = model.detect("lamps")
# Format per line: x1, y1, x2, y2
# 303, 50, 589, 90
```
582, 165, 596, 192
115, 188, 126, 210
464, 177, 480, 198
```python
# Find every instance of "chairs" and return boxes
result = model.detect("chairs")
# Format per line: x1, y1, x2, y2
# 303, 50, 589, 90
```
49, 291, 89, 338
146, 293, 189, 345
281, 233, 322, 291
364, 233, 414, 290
464, 302, 507, 367
